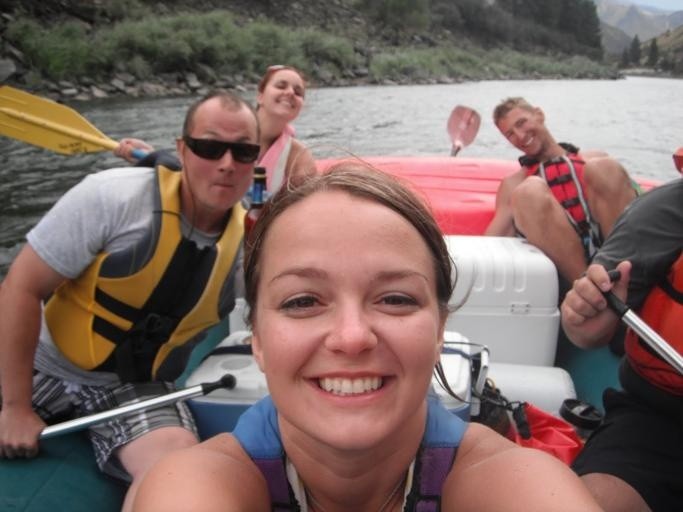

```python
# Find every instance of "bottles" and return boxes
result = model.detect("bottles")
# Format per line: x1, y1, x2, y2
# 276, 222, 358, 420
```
244, 167, 267, 246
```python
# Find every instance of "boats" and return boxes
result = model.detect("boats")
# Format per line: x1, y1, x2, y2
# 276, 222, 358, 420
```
0, 157, 667, 512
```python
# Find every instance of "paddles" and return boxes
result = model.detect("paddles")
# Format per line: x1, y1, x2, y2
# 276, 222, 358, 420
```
0, 85, 272, 205
448, 105, 481, 156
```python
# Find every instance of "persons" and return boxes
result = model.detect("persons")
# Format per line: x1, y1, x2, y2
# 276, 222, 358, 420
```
0, 90, 261, 512
133, 144, 604, 512
483, 97, 636, 286
113, 65, 317, 298
560, 151, 683, 512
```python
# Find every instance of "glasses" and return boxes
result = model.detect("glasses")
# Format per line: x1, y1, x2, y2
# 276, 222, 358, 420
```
184, 136, 260, 163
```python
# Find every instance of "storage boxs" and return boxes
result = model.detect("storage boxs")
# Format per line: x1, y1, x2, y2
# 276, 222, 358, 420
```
184, 327, 472, 444
